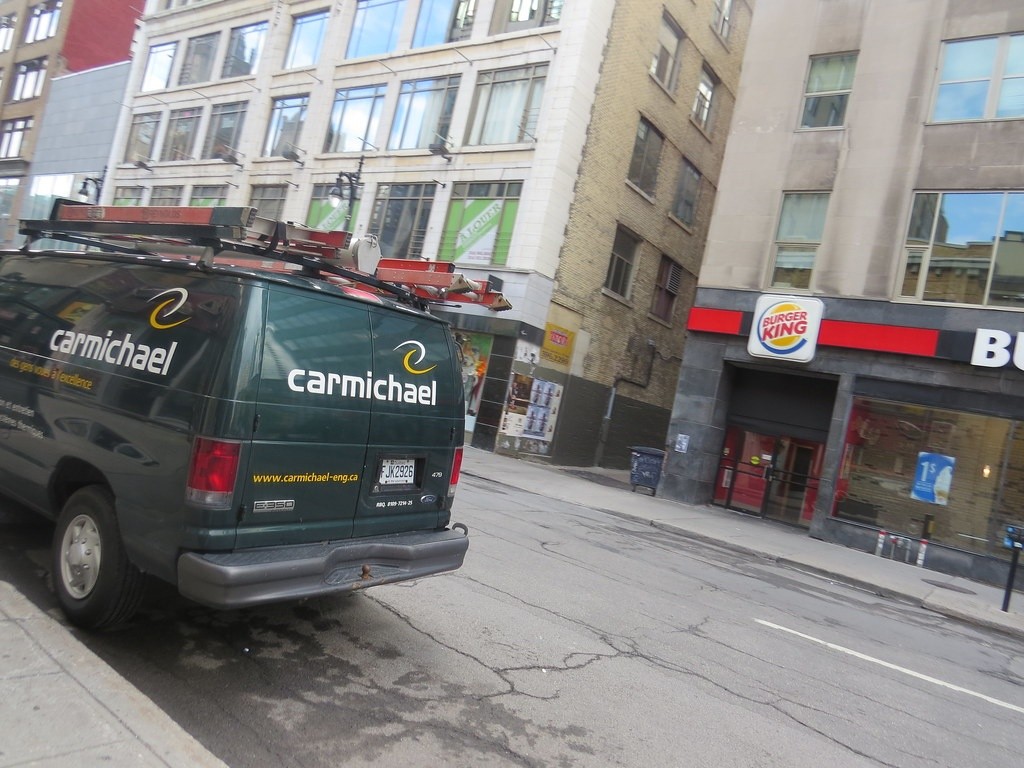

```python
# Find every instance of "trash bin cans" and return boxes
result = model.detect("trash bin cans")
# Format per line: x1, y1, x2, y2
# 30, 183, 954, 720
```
626, 445, 665, 496
837, 493, 883, 524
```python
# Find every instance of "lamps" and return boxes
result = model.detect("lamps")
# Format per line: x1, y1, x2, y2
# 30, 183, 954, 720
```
134, 160, 153, 171
429, 144, 451, 162
222, 154, 243, 168
281, 151, 304, 167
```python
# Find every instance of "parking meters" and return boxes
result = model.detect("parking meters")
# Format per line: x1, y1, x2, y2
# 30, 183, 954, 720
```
998, 526, 1024, 613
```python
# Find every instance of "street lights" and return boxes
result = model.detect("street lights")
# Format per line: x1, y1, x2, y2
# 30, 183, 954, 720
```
328, 171, 356, 254
75, 163, 108, 252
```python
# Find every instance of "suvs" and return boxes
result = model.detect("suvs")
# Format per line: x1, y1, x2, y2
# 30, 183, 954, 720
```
0, 196, 513, 642
